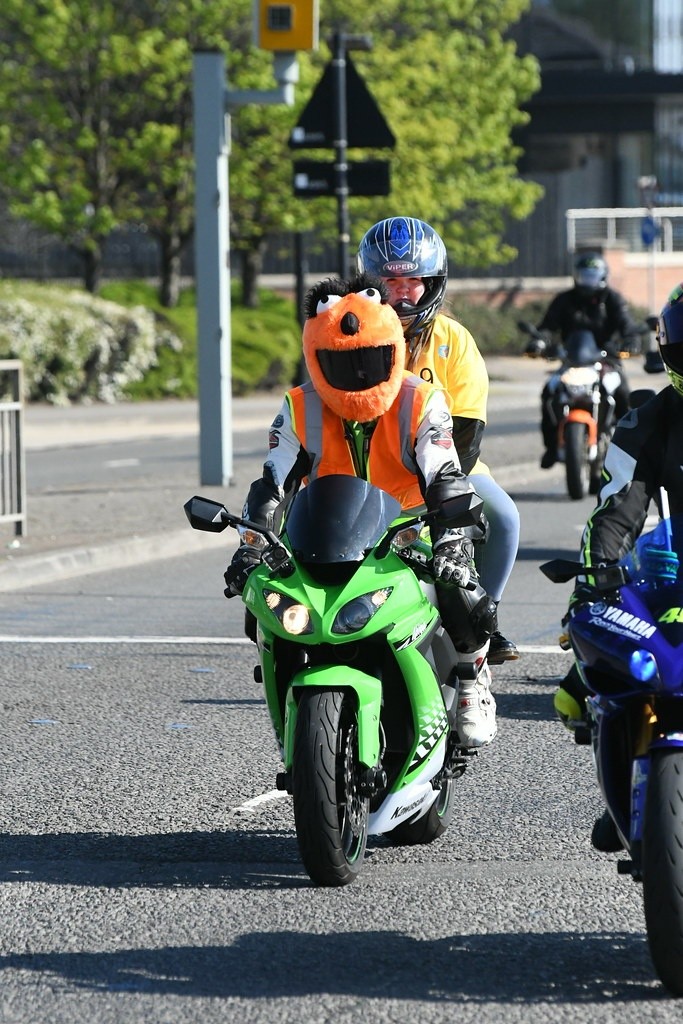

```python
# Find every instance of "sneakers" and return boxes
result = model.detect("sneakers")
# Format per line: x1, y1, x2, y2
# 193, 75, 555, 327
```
455, 658, 499, 749
486, 628, 521, 663
591, 806, 630, 852
540, 446, 558, 470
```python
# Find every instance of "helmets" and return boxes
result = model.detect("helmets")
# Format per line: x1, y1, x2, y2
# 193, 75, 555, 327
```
653, 283, 683, 396
301, 273, 407, 426
573, 251, 609, 293
353, 216, 449, 342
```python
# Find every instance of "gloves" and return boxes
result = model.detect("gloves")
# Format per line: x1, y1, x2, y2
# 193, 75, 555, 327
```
527, 336, 547, 359
431, 528, 482, 589
221, 542, 263, 597
619, 336, 642, 356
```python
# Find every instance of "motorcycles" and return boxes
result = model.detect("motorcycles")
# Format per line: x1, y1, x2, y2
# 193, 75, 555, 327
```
182, 484, 486, 887
537, 546, 681, 1000
514, 315, 658, 502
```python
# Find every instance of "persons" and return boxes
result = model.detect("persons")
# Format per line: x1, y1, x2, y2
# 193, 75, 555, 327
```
323, 217, 524, 662
570, 287, 683, 854
526, 251, 641, 468
225, 292, 498, 748
